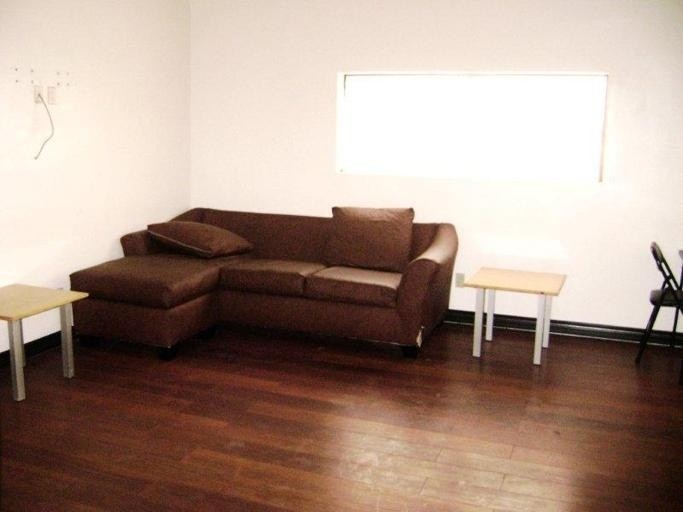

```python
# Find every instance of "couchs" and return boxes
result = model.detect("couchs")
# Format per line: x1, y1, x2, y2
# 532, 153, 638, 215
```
69, 207, 458, 361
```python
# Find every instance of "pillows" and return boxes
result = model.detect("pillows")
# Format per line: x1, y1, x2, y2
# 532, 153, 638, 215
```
320, 207, 415, 273
147, 220, 256, 259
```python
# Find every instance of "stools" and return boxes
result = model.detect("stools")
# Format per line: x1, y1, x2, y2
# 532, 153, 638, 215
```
463, 267, 567, 366
0, 283, 90, 401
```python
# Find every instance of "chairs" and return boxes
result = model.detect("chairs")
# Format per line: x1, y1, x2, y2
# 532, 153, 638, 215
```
635, 242, 683, 386
